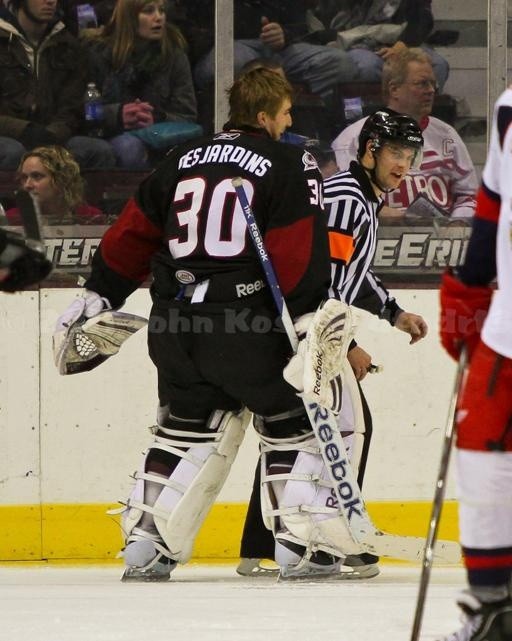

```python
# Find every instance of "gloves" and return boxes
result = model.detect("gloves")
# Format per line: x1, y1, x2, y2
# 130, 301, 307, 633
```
440, 272, 492, 364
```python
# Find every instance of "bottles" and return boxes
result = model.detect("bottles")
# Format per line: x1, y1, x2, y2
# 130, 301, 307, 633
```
84, 82, 105, 140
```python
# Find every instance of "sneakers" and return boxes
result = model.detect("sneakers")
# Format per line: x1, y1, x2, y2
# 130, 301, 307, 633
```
275, 532, 341, 565
125, 528, 176, 564
436, 591, 511, 639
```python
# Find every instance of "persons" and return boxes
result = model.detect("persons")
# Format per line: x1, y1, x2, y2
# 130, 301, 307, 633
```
235, 107, 430, 566
305, 0, 450, 95
0, 1, 117, 169
438, 82, 512, 641
331, 47, 479, 256
52, 69, 380, 574
166, 0, 357, 114
6, 144, 105, 224
77, 1, 204, 169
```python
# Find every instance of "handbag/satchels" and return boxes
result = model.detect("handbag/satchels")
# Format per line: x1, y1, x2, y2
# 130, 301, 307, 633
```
134, 122, 203, 159
327, 23, 405, 52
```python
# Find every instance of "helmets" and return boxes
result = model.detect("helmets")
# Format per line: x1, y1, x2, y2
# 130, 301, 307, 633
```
358, 109, 423, 154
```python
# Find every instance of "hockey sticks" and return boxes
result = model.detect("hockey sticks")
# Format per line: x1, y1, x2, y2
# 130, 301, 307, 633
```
231, 177, 462, 568
